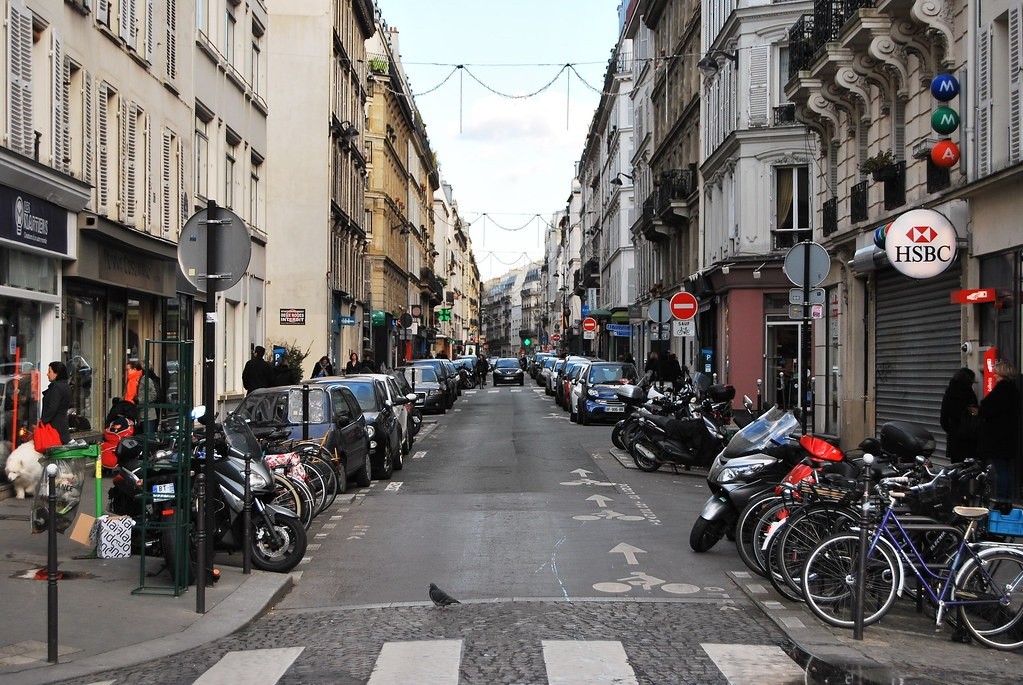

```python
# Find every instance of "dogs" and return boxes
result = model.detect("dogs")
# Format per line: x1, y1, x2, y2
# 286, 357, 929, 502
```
4, 439, 47, 500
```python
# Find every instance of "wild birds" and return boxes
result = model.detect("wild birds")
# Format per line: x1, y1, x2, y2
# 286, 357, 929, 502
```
428, 583, 462, 611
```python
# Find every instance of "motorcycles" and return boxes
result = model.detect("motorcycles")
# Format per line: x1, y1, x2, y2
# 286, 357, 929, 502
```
612, 352, 802, 474
689, 394, 938, 555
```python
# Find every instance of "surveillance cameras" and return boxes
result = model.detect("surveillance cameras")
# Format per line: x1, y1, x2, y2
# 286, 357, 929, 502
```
961, 342, 973, 354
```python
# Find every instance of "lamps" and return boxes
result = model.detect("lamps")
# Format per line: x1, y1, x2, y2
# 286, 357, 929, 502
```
559, 285, 569, 291
391, 221, 412, 236
587, 226, 603, 235
446, 270, 456, 275
328, 117, 359, 137
553, 270, 564, 278
425, 248, 439, 255
611, 173, 634, 185
696, 48, 739, 77
689, 264, 716, 281
722, 263, 736, 274
753, 262, 767, 279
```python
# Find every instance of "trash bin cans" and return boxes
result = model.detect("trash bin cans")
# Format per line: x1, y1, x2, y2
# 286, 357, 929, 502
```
30, 444, 99, 534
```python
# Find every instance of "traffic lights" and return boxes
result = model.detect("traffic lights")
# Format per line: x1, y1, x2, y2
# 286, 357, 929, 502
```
524, 338, 531, 346
451, 340, 456, 345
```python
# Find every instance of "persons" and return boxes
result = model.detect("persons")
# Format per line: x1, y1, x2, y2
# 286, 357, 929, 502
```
970, 359, 1023, 514
110, 417, 128, 433
310, 356, 334, 379
242, 345, 273, 395
32, 362, 72, 446
474, 354, 489, 385
645, 351, 681, 382
361, 355, 375, 371
624, 353, 635, 366
124, 360, 161, 435
346, 353, 361, 374
939, 367, 978, 499
435, 350, 448, 359
272, 358, 295, 387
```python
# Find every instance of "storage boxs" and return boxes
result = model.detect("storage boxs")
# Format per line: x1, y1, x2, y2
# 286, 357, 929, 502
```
710, 383, 735, 402
71, 513, 134, 559
615, 384, 644, 403
988, 509, 1023, 536
879, 421, 937, 463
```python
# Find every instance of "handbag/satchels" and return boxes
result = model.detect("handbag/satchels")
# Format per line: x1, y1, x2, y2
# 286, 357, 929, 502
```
33, 420, 62, 453
132, 396, 140, 405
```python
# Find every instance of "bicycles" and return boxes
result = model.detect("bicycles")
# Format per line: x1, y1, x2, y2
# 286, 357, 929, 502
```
108, 391, 340, 572
733, 436, 1023, 653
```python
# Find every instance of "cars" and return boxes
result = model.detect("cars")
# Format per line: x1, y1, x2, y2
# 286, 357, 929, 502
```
492, 358, 525, 386
298, 377, 404, 480
338, 355, 477, 455
522, 352, 646, 426
224, 384, 370, 495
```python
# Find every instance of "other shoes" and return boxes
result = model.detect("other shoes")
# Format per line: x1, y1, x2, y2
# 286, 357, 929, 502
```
483, 381, 487, 385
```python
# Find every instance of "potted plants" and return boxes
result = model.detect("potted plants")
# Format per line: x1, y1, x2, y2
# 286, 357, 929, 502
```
857, 148, 896, 180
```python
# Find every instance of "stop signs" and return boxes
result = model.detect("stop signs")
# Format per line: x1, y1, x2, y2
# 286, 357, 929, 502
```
553, 334, 560, 341
582, 317, 596, 332
670, 292, 698, 321
